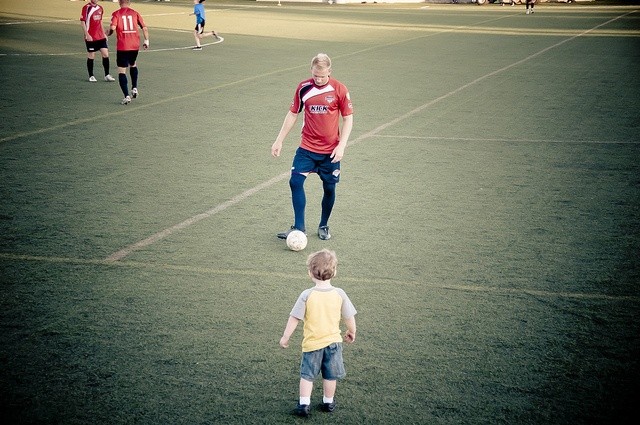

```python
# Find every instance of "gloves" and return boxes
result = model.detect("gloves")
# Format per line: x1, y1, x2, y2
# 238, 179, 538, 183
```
143, 40, 149, 49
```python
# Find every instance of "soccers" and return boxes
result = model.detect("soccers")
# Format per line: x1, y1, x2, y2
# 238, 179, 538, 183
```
286, 231, 307, 251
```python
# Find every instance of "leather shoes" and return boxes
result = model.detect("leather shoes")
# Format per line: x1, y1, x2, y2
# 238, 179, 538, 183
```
321, 401, 336, 412
289, 405, 311, 417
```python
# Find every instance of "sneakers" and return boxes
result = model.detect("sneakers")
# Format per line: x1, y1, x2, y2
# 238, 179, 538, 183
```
277, 226, 307, 239
89, 77, 97, 82
131, 88, 138, 99
212, 30, 218, 39
105, 75, 115, 81
193, 47, 202, 50
318, 225, 331, 240
121, 95, 131, 105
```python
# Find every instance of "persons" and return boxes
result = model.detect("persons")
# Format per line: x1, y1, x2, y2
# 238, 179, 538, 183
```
79, 0, 115, 82
270, 54, 354, 240
188, 0, 218, 51
279, 249, 358, 416
104, 0, 150, 106
525, 0, 536, 14
